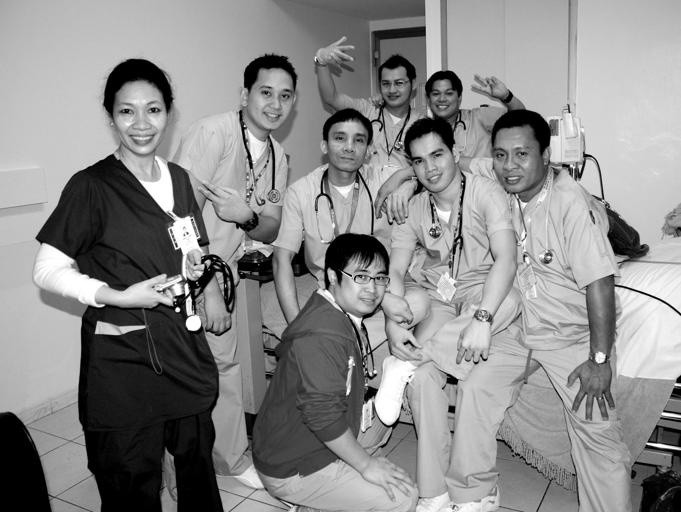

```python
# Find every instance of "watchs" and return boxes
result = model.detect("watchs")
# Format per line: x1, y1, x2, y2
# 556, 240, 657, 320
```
588, 352, 611, 365
313, 57, 327, 68
240, 212, 259, 232
473, 309, 492, 324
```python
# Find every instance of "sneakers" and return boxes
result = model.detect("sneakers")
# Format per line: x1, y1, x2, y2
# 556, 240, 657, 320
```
438, 484, 501, 512
374, 353, 408, 425
414, 492, 449, 512
233, 462, 265, 490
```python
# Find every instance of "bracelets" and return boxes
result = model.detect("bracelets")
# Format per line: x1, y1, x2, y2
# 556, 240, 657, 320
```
502, 90, 514, 105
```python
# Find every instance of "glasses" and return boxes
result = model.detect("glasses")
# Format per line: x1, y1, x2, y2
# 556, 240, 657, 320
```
381, 78, 410, 88
340, 270, 391, 287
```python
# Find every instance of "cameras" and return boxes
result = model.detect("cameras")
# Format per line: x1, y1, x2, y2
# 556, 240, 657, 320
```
150, 273, 187, 305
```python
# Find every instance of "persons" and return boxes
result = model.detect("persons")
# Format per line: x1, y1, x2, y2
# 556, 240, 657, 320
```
252, 232, 419, 512
359, 118, 520, 511
271, 107, 431, 359
425, 70, 526, 158
33, 58, 231, 511
374, 110, 635, 511
315, 36, 430, 168
169, 54, 297, 502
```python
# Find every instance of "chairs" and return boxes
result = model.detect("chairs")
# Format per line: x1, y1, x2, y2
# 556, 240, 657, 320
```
0, 410, 52, 511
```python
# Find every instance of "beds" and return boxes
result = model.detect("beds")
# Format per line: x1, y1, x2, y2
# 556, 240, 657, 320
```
235, 237, 681, 480
